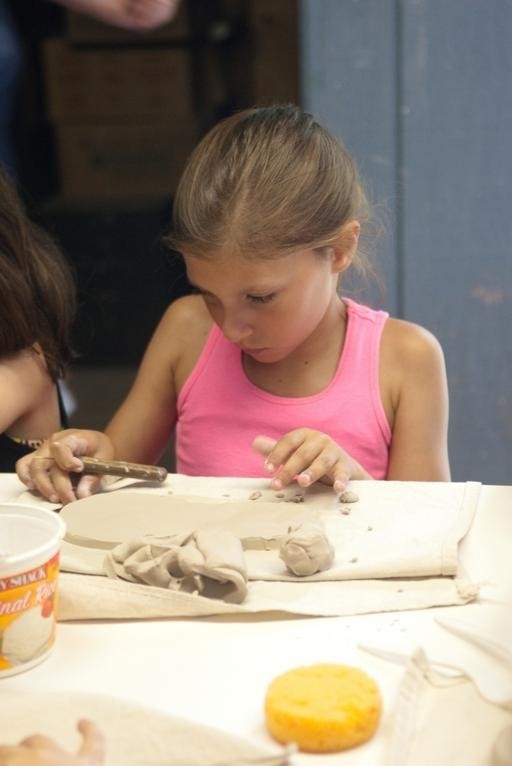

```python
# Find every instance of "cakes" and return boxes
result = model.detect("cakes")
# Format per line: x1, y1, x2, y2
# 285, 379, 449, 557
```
2, 597, 55, 667
264, 667, 382, 753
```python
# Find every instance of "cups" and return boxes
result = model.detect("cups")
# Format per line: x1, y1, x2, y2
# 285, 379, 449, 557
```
0, 501, 66, 679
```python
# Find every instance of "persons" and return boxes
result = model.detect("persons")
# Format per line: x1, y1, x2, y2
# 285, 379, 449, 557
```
0, 2, 180, 178
0, 177, 78, 473
15, 102, 451, 506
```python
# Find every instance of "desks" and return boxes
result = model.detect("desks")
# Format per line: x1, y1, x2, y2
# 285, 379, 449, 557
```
0, 462, 511, 764
